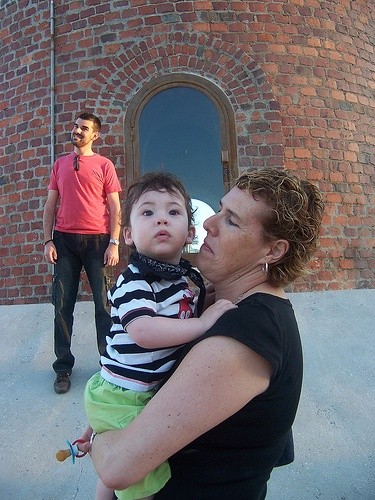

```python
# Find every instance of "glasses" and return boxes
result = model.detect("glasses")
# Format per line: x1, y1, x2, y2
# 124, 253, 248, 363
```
73, 155, 80, 171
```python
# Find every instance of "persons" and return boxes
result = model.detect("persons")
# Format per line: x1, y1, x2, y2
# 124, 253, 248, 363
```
73, 165, 322, 500
86, 172, 238, 500
39, 112, 124, 396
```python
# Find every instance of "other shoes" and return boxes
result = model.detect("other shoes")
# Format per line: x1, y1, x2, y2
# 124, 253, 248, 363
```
54, 371, 71, 394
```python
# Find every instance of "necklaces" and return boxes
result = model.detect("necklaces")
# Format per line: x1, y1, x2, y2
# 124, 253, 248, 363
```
230, 293, 245, 307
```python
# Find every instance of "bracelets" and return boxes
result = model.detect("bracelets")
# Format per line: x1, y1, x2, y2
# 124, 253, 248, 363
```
88, 431, 96, 449
42, 239, 53, 246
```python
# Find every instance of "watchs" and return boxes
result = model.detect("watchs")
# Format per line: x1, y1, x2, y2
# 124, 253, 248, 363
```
109, 238, 120, 245
